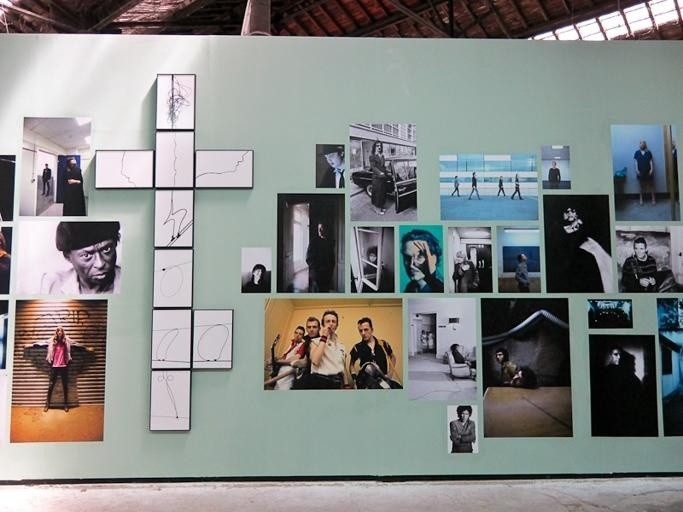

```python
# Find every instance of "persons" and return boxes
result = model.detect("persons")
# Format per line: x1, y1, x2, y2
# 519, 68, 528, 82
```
452, 251, 479, 294
634, 140, 656, 206
23, 327, 95, 413
426, 330, 435, 353
450, 344, 476, 370
451, 176, 459, 197
401, 229, 443, 293
244, 264, 270, 293
591, 305, 628, 326
510, 174, 522, 200
450, 406, 475, 453
291, 317, 320, 389
305, 221, 334, 293
41, 164, 52, 197
40, 222, 121, 295
272, 326, 304, 390
309, 311, 354, 389
348, 317, 400, 389
316, 144, 345, 188
622, 237, 657, 293
420, 330, 428, 353
369, 141, 386, 215
562, 203, 612, 294
548, 161, 560, 189
514, 253, 531, 294
602, 345, 639, 437
0, 232, 9, 295
363, 247, 385, 293
59, 158, 85, 216
495, 349, 517, 385
497, 176, 505, 197
510, 366, 537, 390
469, 172, 480, 200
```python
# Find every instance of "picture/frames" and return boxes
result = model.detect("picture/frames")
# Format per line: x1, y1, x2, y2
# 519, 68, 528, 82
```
192, 309, 232, 370
153, 249, 193, 308
157, 73, 194, 131
196, 150, 254, 188
154, 190, 195, 249
94, 151, 153, 191
154, 132, 194, 188
149, 372, 191, 432
151, 310, 193, 371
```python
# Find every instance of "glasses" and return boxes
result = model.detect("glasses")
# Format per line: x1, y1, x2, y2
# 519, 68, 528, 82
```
376, 144, 382, 147
496, 354, 504, 356
563, 210, 576, 213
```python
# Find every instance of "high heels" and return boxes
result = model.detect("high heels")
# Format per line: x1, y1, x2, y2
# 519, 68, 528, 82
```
652, 200, 656, 206
640, 200, 644, 207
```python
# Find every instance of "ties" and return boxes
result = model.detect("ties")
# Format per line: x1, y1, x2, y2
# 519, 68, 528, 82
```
339, 169, 344, 188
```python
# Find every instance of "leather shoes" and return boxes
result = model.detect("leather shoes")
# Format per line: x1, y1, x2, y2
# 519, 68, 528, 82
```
382, 208, 386, 212
44, 404, 49, 411
64, 406, 68, 411
375, 209, 385, 215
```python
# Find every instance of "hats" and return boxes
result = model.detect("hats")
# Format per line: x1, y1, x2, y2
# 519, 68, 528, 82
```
56, 221, 120, 250
320, 146, 344, 155
